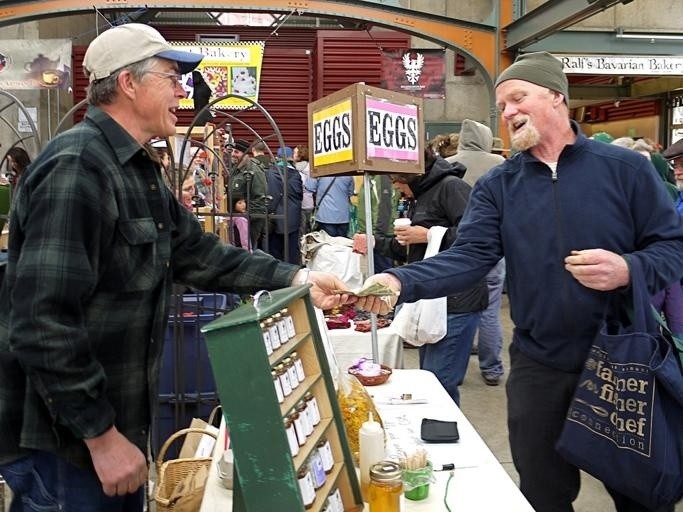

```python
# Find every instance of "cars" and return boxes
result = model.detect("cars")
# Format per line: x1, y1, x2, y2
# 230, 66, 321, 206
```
394, 217, 412, 244
402, 462, 434, 502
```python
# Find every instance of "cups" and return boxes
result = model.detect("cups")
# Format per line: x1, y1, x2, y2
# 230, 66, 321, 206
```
114, 67, 180, 89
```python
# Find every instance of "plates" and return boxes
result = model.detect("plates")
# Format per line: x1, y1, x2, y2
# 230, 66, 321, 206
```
200, 282, 364, 512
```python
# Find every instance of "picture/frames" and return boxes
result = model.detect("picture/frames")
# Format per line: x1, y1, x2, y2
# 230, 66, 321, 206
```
321, 310, 403, 377
198, 367, 534, 512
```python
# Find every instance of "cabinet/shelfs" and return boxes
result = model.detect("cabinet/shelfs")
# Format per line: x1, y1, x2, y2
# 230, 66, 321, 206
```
357, 413, 385, 502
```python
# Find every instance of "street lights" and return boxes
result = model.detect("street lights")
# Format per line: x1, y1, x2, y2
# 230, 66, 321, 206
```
553, 317, 683, 511
310, 206, 320, 231
263, 211, 277, 235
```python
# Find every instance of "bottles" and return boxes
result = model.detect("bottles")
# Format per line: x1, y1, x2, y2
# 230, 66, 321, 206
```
231, 139, 252, 154
493, 51, 569, 108
277, 147, 295, 163
662, 138, 683, 161
82, 23, 204, 86
491, 137, 511, 152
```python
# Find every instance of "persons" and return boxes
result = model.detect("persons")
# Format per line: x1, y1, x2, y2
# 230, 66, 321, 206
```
350, 173, 419, 350
490, 137, 511, 156
355, 51, 683, 512
148, 137, 363, 269
432, 133, 460, 159
6, 147, 33, 231
0, 19, 355, 512
583, 131, 682, 344
444, 119, 504, 386
352, 140, 489, 409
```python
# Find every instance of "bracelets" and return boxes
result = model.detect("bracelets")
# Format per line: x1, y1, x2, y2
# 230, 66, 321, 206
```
348, 364, 391, 387
